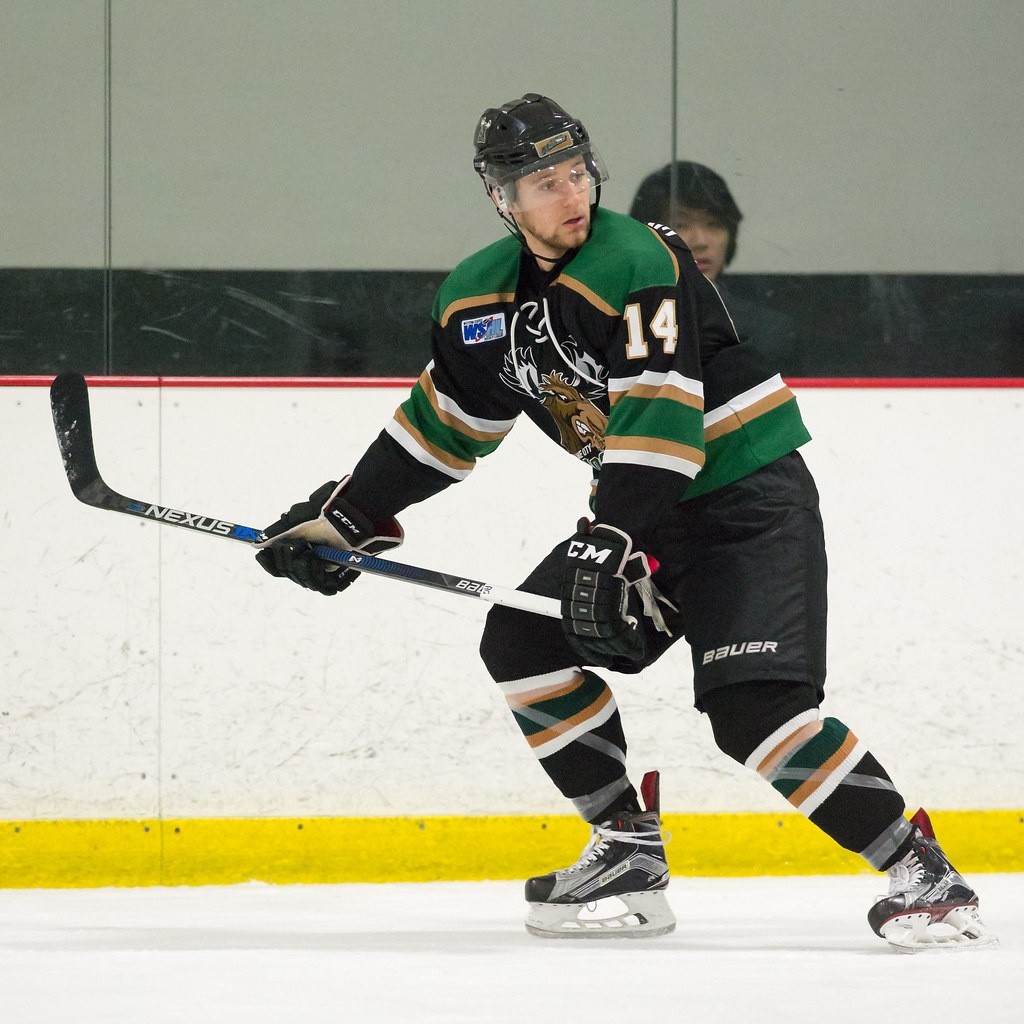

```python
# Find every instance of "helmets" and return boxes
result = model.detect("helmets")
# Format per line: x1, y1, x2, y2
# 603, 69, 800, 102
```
473, 92, 593, 188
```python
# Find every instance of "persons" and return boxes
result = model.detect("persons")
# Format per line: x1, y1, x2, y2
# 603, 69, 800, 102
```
252, 94, 998, 952
632, 160, 798, 377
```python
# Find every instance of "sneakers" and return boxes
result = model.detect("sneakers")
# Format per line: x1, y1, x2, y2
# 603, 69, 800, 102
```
867, 805, 999, 953
525, 769, 677, 938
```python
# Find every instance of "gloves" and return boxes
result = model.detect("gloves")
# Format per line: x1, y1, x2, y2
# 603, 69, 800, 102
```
251, 474, 404, 597
561, 517, 680, 675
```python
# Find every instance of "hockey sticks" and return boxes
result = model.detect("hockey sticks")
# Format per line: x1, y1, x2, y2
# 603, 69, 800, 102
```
47, 370, 638, 634
